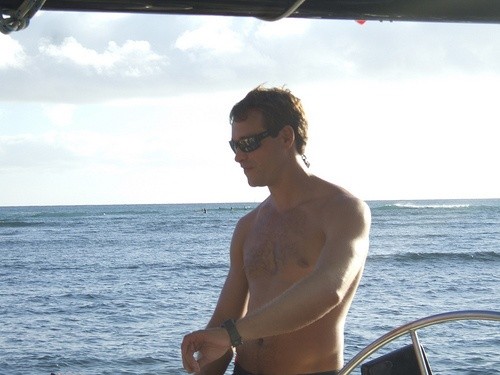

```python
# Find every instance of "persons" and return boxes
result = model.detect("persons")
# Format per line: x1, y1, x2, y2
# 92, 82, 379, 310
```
180, 86, 372, 375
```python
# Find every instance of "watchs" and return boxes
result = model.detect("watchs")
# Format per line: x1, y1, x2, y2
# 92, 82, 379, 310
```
219, 318, 243, 348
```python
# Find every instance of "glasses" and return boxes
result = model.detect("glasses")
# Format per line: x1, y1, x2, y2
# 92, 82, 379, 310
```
229, 131, 272, 154
